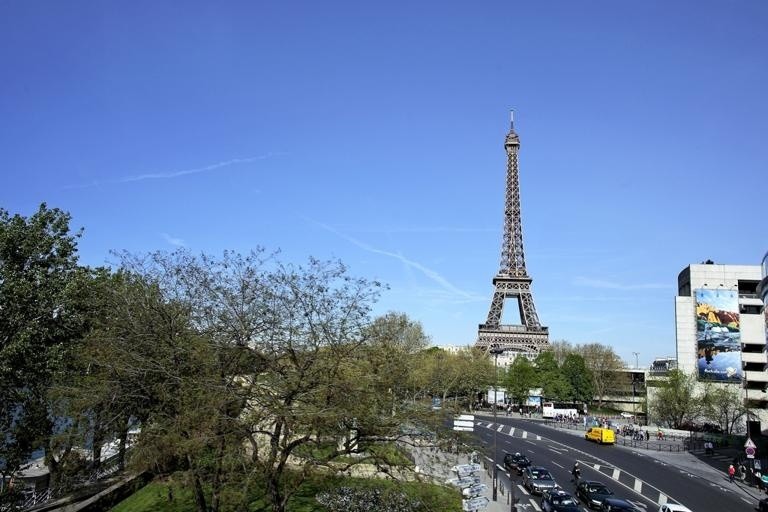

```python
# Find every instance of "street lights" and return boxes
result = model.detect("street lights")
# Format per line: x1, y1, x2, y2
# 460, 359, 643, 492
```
488, 340, 503, 502
631, 351, 641, 369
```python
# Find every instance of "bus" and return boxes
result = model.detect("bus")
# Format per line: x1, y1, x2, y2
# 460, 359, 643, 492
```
541, 401, 588, 420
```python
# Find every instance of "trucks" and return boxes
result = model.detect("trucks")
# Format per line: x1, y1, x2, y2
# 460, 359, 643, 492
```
585, 427, 616, 444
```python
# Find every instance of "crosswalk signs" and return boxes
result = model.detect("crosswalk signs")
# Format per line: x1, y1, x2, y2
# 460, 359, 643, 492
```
753, 460, 760, 469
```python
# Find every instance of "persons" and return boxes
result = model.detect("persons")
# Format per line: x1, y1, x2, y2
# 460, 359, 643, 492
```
572, 461, 582, 485
472, 400, 540, 420
590, 415, 612, 430
727, 464, 735, 482
657, 430, 664, 440
704, 441, 709, 454
617, 422, 650, 441
737, 462, 747, 481
707, 441, 714, 455
554, 411, 579, 427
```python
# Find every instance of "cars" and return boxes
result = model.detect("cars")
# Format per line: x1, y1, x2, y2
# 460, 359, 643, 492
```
503, 451, 642, 511
657, 503, 692, 512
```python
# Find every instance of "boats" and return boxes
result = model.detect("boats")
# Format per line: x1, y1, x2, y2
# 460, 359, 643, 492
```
12, 421, 143, 489
